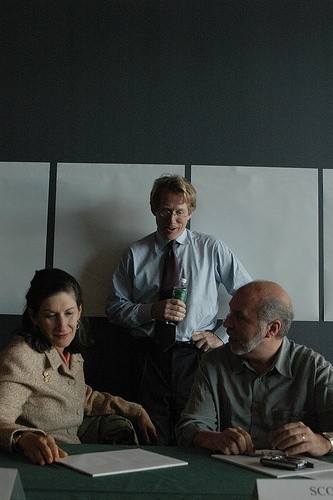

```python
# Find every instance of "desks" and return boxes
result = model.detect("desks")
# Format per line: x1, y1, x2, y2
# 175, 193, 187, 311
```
0, 443, 333, 500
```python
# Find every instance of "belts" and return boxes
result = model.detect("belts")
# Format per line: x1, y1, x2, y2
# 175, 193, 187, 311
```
176, 340, 196, 345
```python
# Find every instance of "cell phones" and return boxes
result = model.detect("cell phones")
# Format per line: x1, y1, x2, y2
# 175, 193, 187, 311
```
261, 454, 314, 471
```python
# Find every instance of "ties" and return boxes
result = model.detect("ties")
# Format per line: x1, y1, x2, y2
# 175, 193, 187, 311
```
152, 240, 179, 353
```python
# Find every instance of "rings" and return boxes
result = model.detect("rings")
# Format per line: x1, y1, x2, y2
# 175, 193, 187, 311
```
300, 434, 306, 443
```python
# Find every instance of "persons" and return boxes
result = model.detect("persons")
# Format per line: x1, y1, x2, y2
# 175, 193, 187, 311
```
105, 173, 254, 446
0, 268, 158, 466
174, 281, 333, 457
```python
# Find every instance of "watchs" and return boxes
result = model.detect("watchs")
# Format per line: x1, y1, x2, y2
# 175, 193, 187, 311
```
319, 432, 333, 455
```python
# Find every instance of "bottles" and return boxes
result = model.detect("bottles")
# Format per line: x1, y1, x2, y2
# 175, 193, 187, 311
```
165, 278, 188, 325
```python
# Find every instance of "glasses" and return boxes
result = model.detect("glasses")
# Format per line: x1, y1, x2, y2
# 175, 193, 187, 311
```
155, 208, 188, 217
